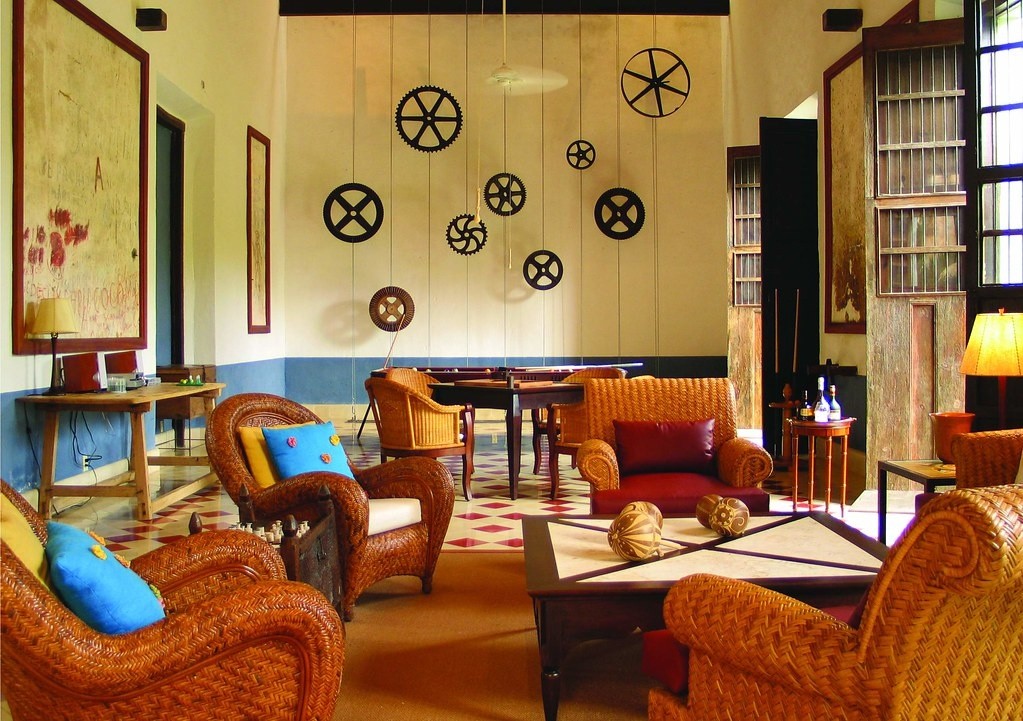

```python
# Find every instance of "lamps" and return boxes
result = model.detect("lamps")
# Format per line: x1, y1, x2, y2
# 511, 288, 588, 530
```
958, 308, 1023, 429
33, 297, 78, 395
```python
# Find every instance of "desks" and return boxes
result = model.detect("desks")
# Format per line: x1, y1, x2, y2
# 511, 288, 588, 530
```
188, 483, 343, 623
373, 365, 583, 498
876, 460, 955, 545
15, 381, 225, 520
786, 417, 855, 516
522, 512, 892, 721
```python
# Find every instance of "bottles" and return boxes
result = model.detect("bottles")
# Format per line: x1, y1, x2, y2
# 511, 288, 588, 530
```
830, 385, 841, 420
796, 390, 813, 421
813, 376, 830, 422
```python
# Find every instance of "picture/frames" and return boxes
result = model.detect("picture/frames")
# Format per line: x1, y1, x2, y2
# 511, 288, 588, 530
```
247, 124, 271, 334
10, 0, 149, 356
823, 0, 919, 335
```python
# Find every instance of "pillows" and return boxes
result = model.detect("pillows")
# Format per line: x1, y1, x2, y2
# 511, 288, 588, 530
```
613, 419, 715, 475
262, 421, 354, 481
238, 419, 317, 490
42, 519, 165, 634
0, 492, 53, 594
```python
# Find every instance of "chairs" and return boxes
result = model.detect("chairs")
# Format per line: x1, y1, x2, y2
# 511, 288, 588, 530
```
364, 376, 474, 501
371, 367, 476, 474
0, 481, 345, 721
639, 483, 1023, 721
204, 394, 455, 625
531, 366, 625, 472
577, 377, 774, 518
950, 429, 1023, 490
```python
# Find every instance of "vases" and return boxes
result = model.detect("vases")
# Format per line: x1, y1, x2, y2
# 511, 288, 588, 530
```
930, 412, 976, 463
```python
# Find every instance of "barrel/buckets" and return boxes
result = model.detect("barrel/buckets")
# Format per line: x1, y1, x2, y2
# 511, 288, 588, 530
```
928, 413, 975, 464
